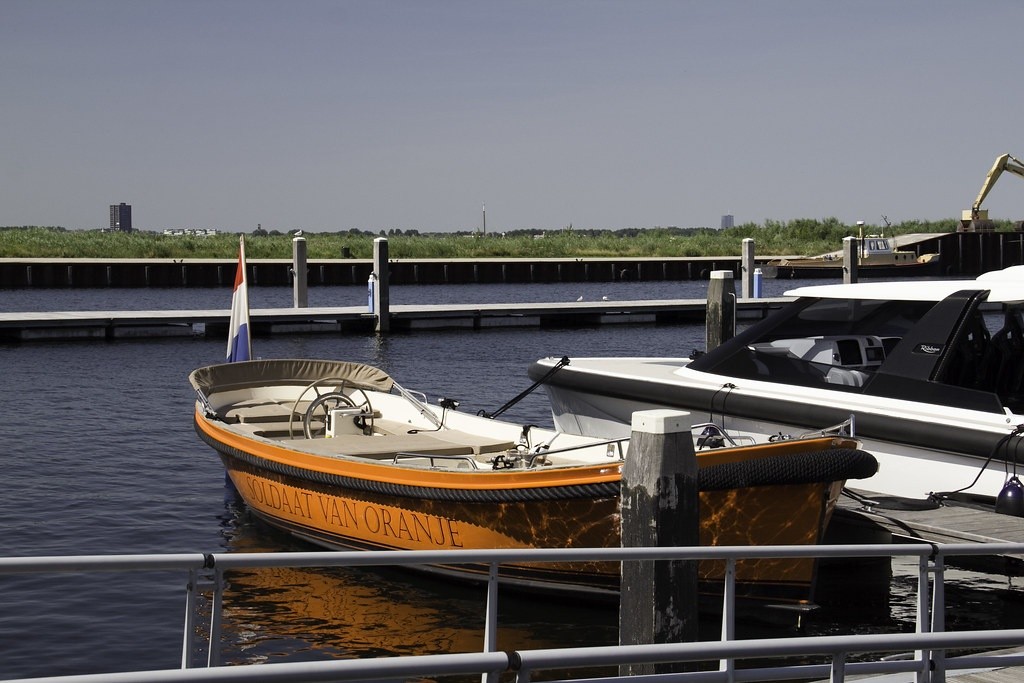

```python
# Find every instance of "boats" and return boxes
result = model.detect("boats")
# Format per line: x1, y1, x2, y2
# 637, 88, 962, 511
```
189, 361, 879, 618
525, 266, 1024, 593
767, 224, 942, 279
205, 529, 605, 679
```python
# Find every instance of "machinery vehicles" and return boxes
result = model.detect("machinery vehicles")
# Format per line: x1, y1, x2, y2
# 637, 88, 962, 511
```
957, 153, 1024, 233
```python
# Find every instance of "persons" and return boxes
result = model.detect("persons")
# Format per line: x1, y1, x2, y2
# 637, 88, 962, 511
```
827, 255, 833, 260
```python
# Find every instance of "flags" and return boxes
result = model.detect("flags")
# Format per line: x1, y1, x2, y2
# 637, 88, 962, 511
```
226, 249, 250, 362
